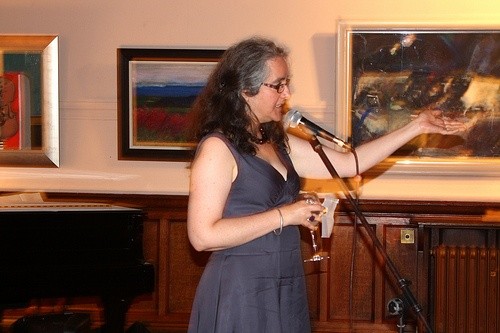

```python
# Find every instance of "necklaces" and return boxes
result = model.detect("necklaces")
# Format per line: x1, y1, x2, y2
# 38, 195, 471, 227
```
246, 126, 267, 144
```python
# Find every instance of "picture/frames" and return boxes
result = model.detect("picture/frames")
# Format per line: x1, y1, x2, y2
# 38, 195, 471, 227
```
336, 21, 500, 177
117, 47, 227, 162
0, 34, 59, 169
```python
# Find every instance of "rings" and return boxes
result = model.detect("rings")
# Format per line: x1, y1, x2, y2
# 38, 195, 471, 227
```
308, 215, 315, 221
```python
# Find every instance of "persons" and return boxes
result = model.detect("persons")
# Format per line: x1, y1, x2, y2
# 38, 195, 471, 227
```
186, 37, 474, 333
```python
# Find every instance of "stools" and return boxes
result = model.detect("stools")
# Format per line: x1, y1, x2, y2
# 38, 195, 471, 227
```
8, 309, 93, 333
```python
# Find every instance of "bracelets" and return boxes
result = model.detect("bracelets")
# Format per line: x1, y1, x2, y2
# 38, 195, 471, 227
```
267, 207, 282, 235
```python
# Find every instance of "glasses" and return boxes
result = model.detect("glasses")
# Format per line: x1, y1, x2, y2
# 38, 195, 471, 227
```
261, 79, 290, 93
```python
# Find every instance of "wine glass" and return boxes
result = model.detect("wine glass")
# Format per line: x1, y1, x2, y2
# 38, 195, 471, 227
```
296, 192, 330, 263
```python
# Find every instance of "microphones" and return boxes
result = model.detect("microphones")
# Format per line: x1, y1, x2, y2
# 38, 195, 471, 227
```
285, 109, 355, 152
